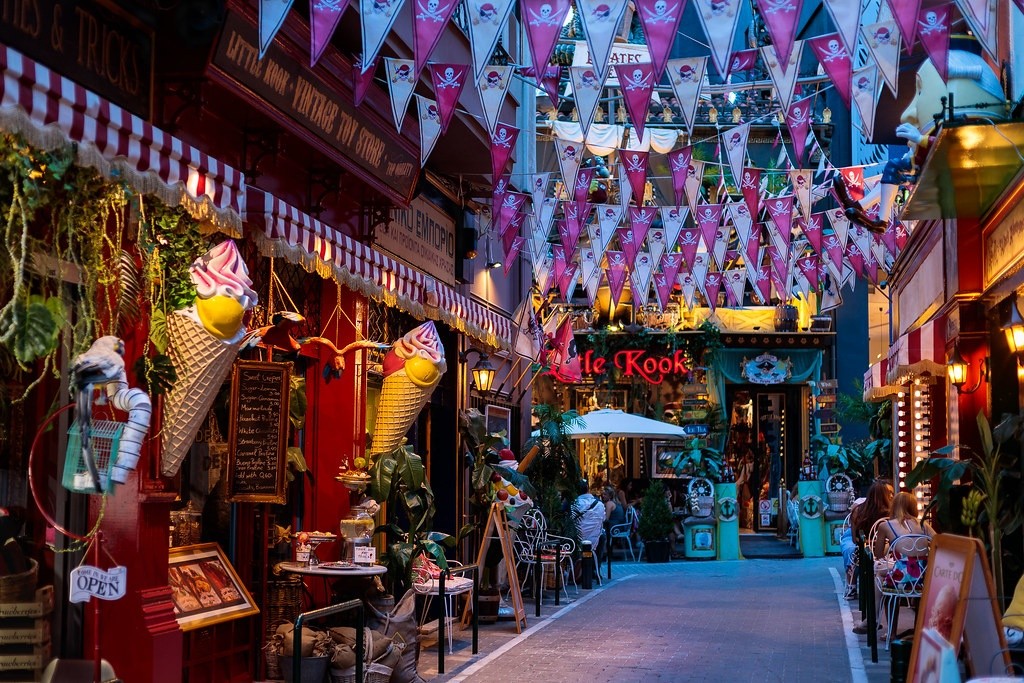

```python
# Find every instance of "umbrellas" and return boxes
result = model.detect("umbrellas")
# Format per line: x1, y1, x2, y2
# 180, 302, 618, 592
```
533, 407, 687, 482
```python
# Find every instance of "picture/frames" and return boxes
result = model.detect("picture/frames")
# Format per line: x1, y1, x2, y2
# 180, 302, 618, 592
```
166, 542, 259, 634
227, 355, 296, 507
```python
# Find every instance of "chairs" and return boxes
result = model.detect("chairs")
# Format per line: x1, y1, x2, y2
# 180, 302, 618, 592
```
398, 531, 474, 656
786, 484, 936, 646
504, 503, 645, 603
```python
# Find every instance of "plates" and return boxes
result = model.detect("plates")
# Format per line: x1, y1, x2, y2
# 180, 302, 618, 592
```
318, 562, 361, 569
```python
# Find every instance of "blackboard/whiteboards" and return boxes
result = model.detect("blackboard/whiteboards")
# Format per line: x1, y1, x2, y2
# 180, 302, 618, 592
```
228, 359, 286, 501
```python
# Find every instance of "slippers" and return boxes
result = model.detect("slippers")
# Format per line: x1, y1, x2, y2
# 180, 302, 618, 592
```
852, 621, 883, 635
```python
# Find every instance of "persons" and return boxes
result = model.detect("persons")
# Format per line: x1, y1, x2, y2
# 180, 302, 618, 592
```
666, 487, 684, 559
840, 479, 936, 640
571, 477, 643, 581
896, 50, 1008, 167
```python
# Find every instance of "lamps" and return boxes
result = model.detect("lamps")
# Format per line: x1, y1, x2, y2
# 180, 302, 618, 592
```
1000, 321, 1024, 367
949, 337, 990, 396
459, 344, 495, 401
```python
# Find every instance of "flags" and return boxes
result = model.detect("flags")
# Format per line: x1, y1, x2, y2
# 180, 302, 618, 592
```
511, 291, 582, 382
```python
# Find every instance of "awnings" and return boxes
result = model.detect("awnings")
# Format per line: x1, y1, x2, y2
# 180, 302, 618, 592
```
863, 314, 946, 401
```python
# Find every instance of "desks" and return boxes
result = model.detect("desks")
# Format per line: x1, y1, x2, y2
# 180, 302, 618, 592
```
279, 562, 389, 627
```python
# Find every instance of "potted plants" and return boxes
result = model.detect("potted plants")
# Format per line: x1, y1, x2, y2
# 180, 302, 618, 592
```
639, 478, 676, 562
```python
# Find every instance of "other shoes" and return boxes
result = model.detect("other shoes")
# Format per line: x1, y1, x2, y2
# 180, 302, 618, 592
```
677, 534, 684, 541
672, 552, 678, 559
880, 632, 896, 642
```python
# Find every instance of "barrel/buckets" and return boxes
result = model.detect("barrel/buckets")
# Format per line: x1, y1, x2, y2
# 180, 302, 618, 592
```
277, 654, 329, 683
774, 305, 798, 332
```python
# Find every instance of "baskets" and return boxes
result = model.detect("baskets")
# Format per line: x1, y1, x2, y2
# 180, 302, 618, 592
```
0, 556, 40, 603
826, 491, 850, 511
689, 496, 714, 517
331, 626, 394, 683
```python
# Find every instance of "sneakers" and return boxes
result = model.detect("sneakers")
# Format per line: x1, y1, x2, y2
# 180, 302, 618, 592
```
842, 585, 857, 600
